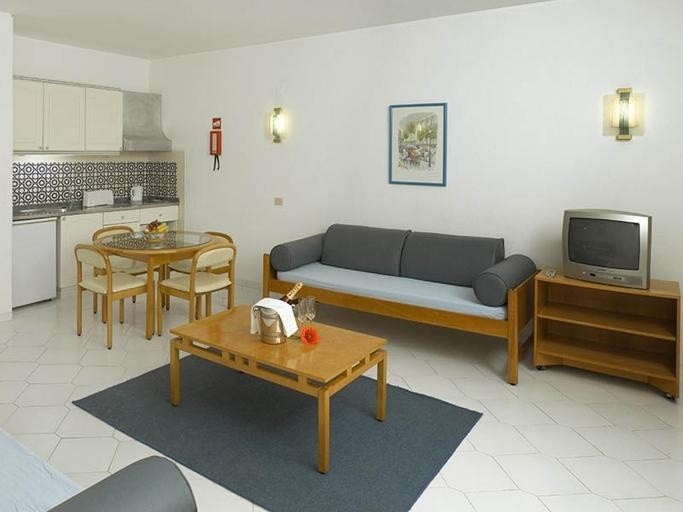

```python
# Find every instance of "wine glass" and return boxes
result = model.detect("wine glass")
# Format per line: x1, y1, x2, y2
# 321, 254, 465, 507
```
295, 299, 320, 341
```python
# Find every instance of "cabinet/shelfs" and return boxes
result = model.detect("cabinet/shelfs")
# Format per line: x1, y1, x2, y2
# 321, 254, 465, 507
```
86, 84, 123, 157
12, 76, 85, 156
56, 212, 103, 300
104, 204, 178, 227
534, 267, 681, 400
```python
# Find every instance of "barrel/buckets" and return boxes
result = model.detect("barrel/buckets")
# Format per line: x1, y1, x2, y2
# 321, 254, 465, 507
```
253, 306, 285, 345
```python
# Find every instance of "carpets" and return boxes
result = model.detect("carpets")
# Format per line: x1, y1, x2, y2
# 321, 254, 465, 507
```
72, 348, 483, 512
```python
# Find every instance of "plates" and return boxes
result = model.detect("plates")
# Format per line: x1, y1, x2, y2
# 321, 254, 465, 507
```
143, 232, 169, 239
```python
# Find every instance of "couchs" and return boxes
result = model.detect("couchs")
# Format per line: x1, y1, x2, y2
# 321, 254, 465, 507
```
262, 253, 541, 386
0, 429, 197, 512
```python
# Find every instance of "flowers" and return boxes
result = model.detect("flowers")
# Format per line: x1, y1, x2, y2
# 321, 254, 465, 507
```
291, 326, 319, 345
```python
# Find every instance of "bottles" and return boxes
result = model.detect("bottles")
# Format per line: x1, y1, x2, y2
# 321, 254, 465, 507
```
279, 281, 304, 303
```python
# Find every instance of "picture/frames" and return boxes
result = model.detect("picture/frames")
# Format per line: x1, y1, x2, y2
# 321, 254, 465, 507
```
389, 103, 446, 187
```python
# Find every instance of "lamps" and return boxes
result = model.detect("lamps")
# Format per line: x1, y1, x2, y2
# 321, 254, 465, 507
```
267, 106, 289, 143
602, 86, 643, 140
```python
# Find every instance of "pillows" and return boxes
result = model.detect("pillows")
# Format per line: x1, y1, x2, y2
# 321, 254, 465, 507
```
399, 230, 504, 287
270, 233, 326, 271
46, 456, 197, 512
471, 254, 536, 307
321, 223, 412, 276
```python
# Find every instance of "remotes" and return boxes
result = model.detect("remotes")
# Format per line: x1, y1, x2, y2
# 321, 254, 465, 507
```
546, 265, 556, 278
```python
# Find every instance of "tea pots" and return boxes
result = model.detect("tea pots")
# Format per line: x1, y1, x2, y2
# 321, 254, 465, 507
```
129, 185, 144, 201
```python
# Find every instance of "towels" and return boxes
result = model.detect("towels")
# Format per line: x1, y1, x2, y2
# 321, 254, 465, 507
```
250, 298, 298, 339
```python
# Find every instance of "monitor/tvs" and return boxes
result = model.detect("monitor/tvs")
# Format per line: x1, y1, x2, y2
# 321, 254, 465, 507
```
560, 208, 652, 291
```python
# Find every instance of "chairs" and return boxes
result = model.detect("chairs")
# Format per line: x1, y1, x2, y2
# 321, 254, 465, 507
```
92, 225, 164, 324
157, 244, 237, 336
75, 244, 155, 350
165, 232, 233, 310
